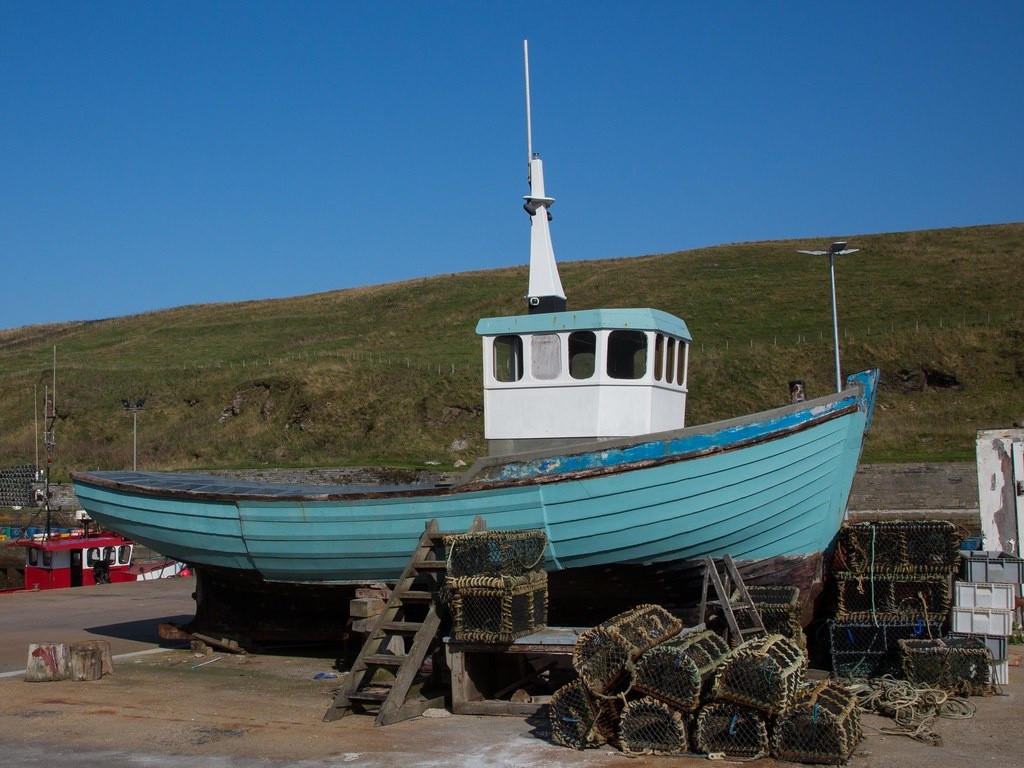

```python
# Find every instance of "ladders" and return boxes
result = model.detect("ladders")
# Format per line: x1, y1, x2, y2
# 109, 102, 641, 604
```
700, 554, 769, 647
323, 515, 488, 727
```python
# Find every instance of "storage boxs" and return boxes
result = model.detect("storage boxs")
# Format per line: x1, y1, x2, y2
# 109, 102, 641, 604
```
948, 550, 1024, 686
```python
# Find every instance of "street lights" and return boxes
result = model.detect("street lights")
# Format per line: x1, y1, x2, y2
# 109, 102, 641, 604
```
121, 398, 147, 469
793, 243, 862, 389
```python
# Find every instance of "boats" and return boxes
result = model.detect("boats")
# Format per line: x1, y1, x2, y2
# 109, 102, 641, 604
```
67, 39, 881, 591
0, 346, 188, 595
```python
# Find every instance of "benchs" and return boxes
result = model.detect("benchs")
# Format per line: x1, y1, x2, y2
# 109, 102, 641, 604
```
442, 626, 592, 717
